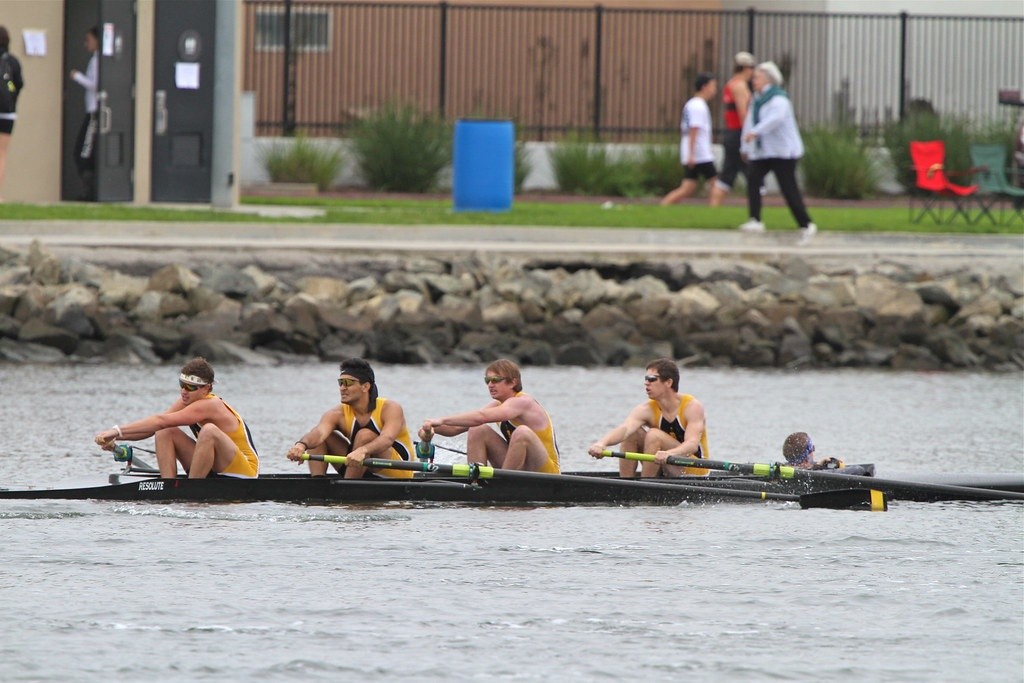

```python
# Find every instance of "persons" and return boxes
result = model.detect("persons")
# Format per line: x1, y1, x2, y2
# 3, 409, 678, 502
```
0, 26, 23, 187
660, 75, 719, 205
709, 50, 768, 207
739, 61, 817, 238
94, 357, 259, 479
68, 26, 99, 202
590, 358, 709, 478
783, 432, 842, 470
417, 358, 561, 474
287, 357, 415, 479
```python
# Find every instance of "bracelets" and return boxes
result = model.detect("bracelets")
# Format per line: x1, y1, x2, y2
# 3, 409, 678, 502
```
295, 441, 308, 451
113, 424, 123, 437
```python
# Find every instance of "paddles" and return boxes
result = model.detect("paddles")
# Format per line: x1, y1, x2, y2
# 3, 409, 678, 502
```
298, 452, 887, 511
600, 449, 1024, 500
417, 422, 434, 463
106, 438, 153, 470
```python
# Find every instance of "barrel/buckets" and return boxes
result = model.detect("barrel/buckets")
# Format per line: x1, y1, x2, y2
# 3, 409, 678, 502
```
451, 119, 515, 211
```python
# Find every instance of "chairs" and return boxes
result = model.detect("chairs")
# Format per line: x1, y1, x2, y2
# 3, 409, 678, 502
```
964, 144, 1024, 227
908, 141, 977, 224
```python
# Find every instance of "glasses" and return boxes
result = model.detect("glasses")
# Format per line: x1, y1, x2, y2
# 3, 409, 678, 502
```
644, 375, 662, 382
179, 379, 206, 391
337, 379, 362, 387
808, 445, 815, 453
484, 376, 515, 384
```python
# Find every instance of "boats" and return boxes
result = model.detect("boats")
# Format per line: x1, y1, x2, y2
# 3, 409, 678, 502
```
0, 471, 1024, 504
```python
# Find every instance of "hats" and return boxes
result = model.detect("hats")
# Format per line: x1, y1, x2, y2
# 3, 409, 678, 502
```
695, 72, 712, 88
758, 60, 783, 86
736, 52, 756, 67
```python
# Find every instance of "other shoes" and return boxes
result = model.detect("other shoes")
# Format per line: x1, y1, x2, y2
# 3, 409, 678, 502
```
739, 217, 765, 232
794, 223, 817, 245
